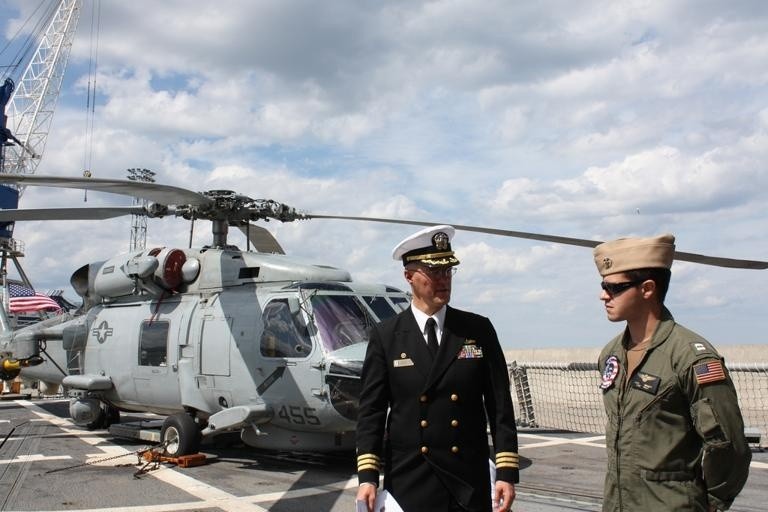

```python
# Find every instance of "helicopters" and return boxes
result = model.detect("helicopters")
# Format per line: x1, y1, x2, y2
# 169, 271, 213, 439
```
0, 174, 768, 458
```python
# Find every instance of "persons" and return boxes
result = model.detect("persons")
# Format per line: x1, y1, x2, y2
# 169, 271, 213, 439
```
587, 229, 754, 512
351, 223, 521, 512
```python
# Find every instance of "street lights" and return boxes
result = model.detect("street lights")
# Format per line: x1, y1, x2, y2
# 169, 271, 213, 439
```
128, 167, 156, 251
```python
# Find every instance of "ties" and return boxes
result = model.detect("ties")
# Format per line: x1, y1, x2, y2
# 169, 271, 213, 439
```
427, 318, 438, 360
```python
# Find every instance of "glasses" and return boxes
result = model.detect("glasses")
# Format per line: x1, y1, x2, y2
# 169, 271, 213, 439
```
409, 266, 457, 277
601, 281, 641, 294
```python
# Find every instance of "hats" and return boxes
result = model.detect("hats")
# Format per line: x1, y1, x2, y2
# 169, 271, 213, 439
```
592, 233, 675, 277
392, 225, 460, 268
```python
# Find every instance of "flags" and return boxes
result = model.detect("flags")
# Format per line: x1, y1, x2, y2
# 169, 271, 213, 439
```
7, 283, 63, 316
